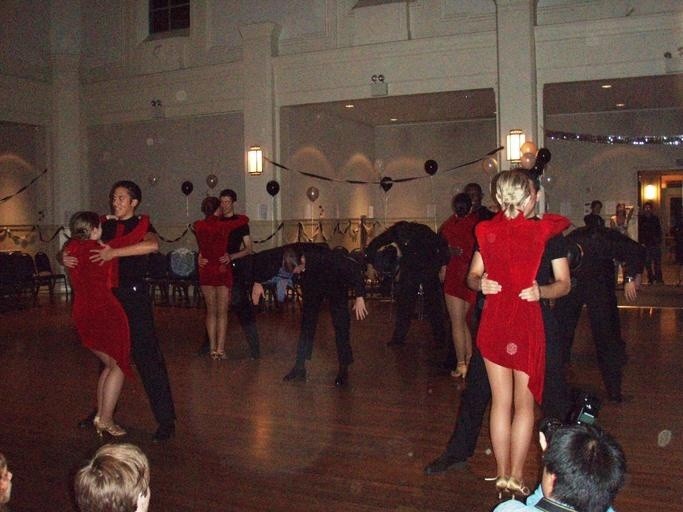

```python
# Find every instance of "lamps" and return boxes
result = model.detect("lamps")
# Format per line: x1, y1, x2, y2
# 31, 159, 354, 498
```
246, 146, 263, 176
506, 129, 526, 162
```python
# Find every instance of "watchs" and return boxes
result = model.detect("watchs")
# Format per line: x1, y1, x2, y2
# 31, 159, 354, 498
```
625, 278, 632, 284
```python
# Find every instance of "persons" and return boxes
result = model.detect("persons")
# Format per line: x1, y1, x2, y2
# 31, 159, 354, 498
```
473, 170, 570, 502
359, 221, 450, 353
582, 200, 604, 227
423, 168, 577, 476
638, 202, 665, 285
56, 180, 177, 444
75, 444, 150, 512
252, 247, 369, 388
610, 203, 634, 289
437, 184, 496, 366
0, 453, 13, 512
439, 194, 487, 380
551, 226, 646, 407
193, 189, 260, 364
493, 423, 625, 512
193, 196, 250, 361
64, 209, 150, 436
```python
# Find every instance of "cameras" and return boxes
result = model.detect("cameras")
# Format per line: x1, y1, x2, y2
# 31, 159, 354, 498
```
539, 393, 604, 445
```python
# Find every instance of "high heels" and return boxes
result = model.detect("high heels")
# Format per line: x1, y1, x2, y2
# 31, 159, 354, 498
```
92, 416, 100, 434
217, 351, 229, 361
209, 350, 217, 360
495, 475, 510, 500
506, 476, 531, 500
97, 420, 128, 437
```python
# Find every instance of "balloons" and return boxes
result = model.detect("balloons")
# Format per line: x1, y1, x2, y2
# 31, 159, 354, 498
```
520, 142, 537, 157
520, 153, 536, 170
206, 175, 219, 189
266, 180, 280, 197
381, 178, 392, 193
425, 160, 437, 175
538, 148, 551, 164
147, 172, 159, 187
451, 183, 465, 198
306, 187, 319, 202
181, 181, 194, 196
483, 157, 498, 176
544, 175, 555, 189
533, 164, 543, 175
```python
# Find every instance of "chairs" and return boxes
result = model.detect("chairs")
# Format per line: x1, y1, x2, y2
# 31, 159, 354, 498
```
35, 251, 69, 299
164, 248, 201, 302
172, 282, 189, 302
350, 247, 374, 296
145, 252, 170, 303
332, 246, 353, 298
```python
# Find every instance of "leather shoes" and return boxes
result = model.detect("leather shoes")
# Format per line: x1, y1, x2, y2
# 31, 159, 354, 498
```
78, 412, 98, 428
424, 453, 467, 475
150, 422, 176, 443
283, 367, 307, 381
334, 373, 346, 385
